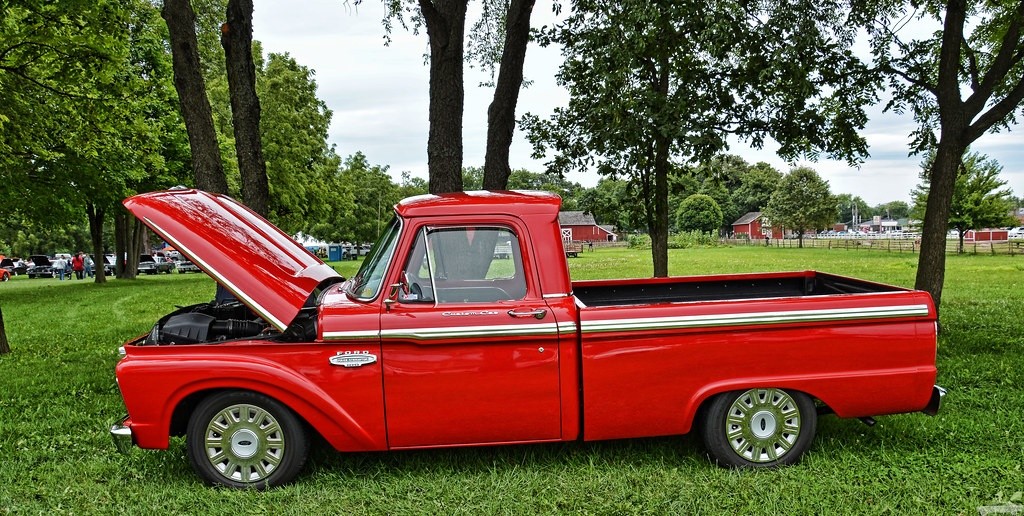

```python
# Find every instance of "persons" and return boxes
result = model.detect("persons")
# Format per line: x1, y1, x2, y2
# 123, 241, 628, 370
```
72, 253, 83, 280
83, 253, 94, 279
57, 255, 66, 280
65, 259, 74, 280
588, 240, 593, 251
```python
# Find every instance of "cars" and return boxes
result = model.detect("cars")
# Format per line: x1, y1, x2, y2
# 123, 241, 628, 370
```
0, 247, 203, 281
302, 244, 373, 259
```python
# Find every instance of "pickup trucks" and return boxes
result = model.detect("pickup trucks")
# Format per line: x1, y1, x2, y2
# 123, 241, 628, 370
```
111, 184, 947, 496
